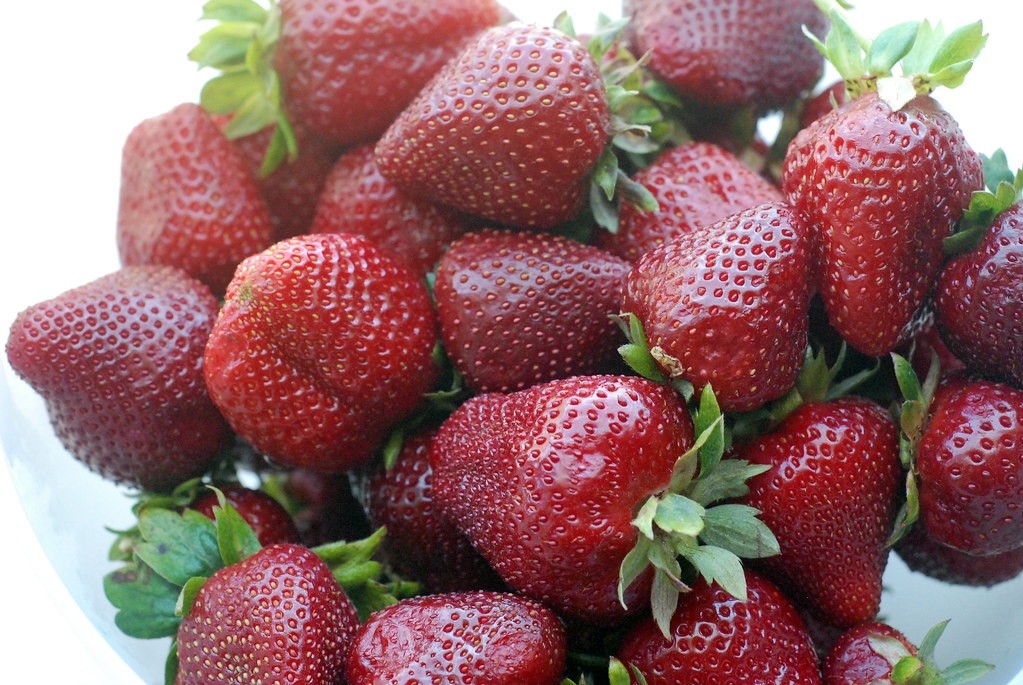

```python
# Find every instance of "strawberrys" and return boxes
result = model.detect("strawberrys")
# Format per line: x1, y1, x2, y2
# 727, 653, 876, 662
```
3, 0, 1022, 684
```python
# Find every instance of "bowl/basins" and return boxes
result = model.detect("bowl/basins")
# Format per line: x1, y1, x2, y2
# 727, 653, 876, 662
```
0, 362, 1023, 685
